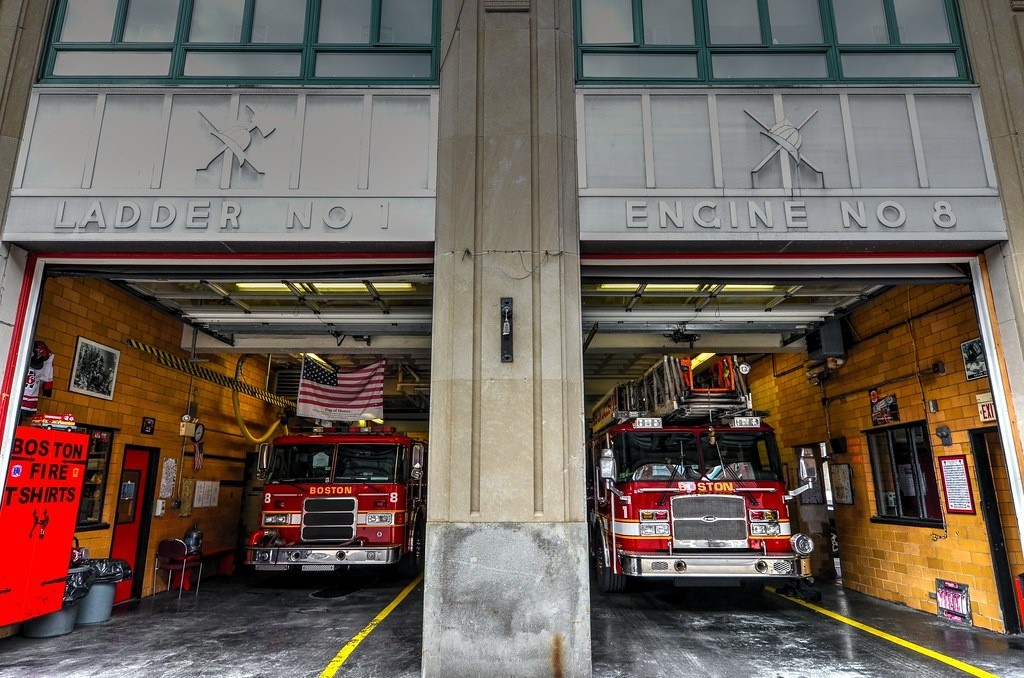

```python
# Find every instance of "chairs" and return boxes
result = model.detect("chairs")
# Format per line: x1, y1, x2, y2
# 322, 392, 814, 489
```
153, 539, 203, 599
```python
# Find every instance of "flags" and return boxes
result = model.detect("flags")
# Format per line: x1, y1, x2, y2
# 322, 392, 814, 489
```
297, 354, 384, 420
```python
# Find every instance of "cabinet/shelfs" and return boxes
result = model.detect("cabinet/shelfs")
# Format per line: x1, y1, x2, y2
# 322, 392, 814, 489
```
0, 425, 90, 628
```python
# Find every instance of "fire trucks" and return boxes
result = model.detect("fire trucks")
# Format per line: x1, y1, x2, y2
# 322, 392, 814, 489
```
586, 355, 816, 598
239, 427, 428, 582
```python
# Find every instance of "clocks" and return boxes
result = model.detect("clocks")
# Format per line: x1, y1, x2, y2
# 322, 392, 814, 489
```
191, 423, 205, 442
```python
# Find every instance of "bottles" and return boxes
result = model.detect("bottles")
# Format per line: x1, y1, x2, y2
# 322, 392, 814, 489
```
184, 523, 204, 555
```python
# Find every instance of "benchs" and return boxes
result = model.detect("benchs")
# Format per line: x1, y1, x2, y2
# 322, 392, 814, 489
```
171, 540, 236, 590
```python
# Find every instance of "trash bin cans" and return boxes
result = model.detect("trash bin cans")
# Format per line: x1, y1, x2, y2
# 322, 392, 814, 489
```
76, 558, 117, 625
21, 564, 91, 638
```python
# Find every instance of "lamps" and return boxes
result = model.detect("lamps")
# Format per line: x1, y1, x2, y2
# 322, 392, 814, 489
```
582, 309, 836, 325
180, 306, 433, 325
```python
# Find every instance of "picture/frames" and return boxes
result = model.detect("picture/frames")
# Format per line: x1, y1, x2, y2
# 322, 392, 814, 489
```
960, 337, 988, 381
140, 417, 156, 435
67, 336, 121, 401
798, 466, 823, 505
829, 463, 854, 506
